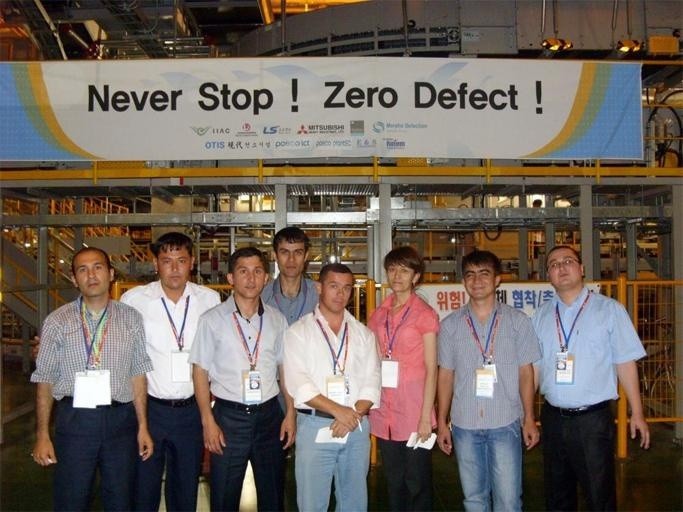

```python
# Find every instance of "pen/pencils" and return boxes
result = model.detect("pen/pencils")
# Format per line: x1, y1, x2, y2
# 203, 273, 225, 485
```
30, 453, 53, 464
413, 438, 422, 450
356, 418, 363, 433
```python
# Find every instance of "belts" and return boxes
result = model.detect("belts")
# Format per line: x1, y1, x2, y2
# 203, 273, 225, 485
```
297, 409, 334, 419
147, 394, 195, 408
543, 399, 610, 413
216, 396, 277, 416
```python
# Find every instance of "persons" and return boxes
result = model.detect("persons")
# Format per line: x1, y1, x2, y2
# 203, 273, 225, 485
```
260, 225, 320, 512
118, 232, 222, 512
437, 250, 543, 512
283, 263, 378, 512
530, 245, 650, 512
30, 247, 153, 512
366, 245, 439, 512
188, 247, 295, 512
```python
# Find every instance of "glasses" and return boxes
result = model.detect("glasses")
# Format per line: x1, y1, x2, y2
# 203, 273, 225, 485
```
546, 257, 579, 271
